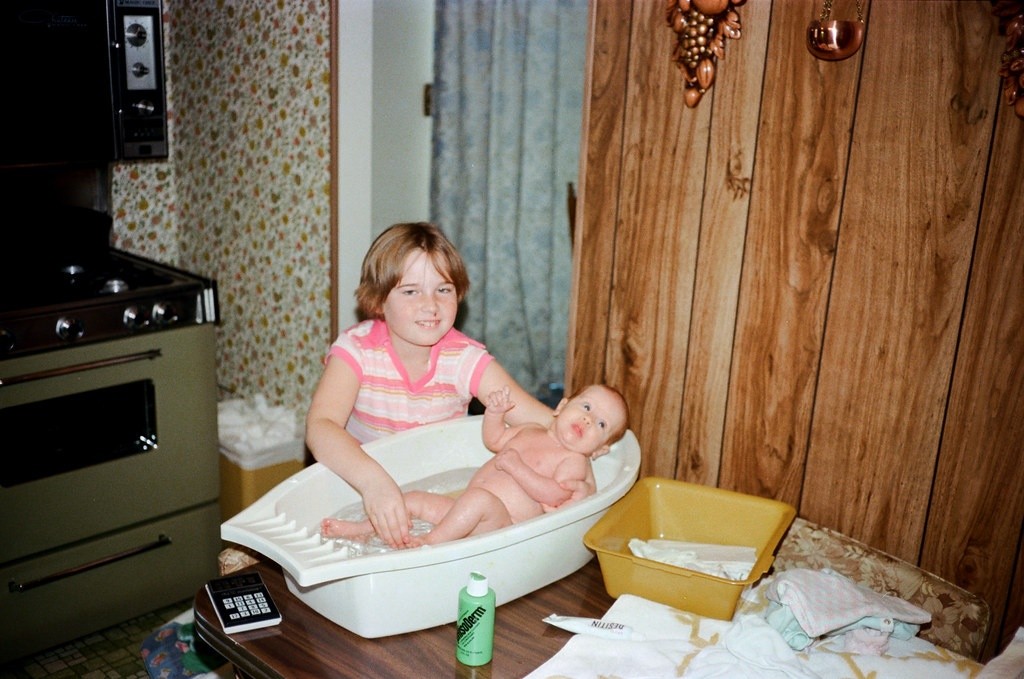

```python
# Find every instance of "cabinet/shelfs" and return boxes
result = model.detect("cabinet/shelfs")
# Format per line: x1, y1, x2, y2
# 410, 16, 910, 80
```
0, 324, 227, 666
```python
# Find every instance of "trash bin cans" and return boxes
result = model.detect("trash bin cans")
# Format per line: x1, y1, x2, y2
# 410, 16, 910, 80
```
218, 396, 306, 521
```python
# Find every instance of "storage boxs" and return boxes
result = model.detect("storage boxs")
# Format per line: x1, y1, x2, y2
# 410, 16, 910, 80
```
583, 477, 798, 623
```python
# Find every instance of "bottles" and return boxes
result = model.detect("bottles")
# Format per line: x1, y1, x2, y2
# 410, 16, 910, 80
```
455, 572, 496, 663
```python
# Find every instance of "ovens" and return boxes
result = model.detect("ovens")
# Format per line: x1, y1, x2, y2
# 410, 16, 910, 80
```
1, 322, 226, 665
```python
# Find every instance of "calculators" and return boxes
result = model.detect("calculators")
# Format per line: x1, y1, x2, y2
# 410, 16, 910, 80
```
208, 570, 283, 634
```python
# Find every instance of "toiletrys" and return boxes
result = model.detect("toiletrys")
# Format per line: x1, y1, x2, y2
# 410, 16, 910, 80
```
540, 613, 650, 648
454, 553, 499, 670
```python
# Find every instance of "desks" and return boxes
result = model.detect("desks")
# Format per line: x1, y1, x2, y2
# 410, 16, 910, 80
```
193, 553, 987, 679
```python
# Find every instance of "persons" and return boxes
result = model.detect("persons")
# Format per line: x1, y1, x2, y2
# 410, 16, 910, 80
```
301, 222, 601, 550
320, 383, 633, 553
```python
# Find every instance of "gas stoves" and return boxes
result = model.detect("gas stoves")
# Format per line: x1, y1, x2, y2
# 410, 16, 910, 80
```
1, 205, 219, 360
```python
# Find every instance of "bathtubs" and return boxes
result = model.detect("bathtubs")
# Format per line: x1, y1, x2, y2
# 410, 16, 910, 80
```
220, 410, 643, 645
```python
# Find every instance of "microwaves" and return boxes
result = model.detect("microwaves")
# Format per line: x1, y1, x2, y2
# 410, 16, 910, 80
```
1, 0, 170, 167
